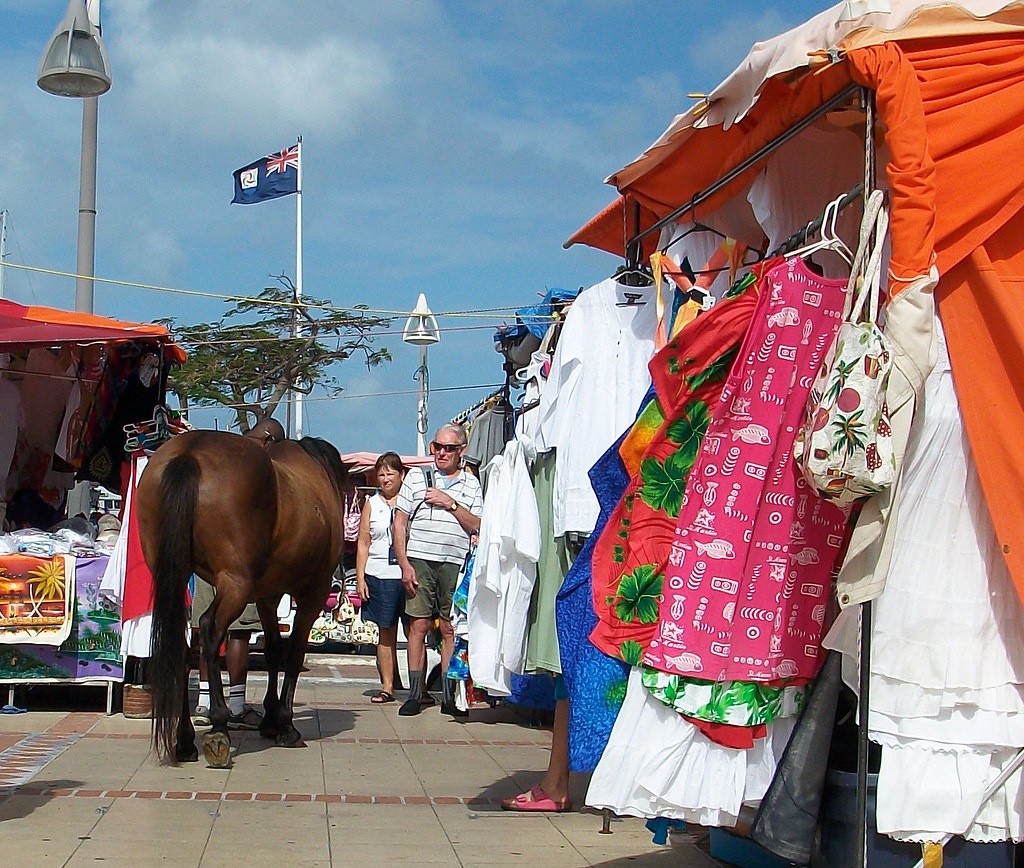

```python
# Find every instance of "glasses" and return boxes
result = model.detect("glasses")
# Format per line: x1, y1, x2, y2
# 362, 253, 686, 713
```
432, 441, 461, 452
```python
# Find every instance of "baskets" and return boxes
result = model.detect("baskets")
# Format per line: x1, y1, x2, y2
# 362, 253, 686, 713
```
123, 683, 200, 719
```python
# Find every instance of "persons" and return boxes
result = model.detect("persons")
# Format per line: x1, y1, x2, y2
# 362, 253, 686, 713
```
392, 423, 486, 717
2, 486, 68, 533
190, 415, 287, 731
500, 672, 574, 814
355, 450, 438, 706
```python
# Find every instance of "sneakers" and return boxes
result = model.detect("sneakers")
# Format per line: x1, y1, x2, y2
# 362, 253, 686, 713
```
227, 704, 263, 731
193, 706, 212, 726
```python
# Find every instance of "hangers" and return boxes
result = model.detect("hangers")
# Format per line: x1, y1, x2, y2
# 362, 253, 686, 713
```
759, 194, 858, 270
642, 191, 765, 275
576, 238, 680, 306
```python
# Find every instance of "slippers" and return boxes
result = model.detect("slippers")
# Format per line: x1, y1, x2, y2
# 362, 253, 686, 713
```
371, 691, 395, 703
500, 785, 572, 811
420, 691, 435, 706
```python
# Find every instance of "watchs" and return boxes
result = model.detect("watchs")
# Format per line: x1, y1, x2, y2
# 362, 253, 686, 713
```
446, 501, 459, 513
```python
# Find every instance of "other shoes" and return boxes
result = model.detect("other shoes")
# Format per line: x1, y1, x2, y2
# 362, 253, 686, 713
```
398, 699, 421, 715
440, 700, 468, 716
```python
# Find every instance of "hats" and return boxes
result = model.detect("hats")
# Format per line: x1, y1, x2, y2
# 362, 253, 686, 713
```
74, 512, 121, 540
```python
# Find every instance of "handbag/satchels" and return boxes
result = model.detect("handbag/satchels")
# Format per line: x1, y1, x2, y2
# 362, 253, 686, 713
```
794, 187, 895, 506
307, 592, 378, 645
343, 486, 361, 542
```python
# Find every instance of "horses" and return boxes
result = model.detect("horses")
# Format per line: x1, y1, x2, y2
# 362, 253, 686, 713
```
134, 428, 348, 767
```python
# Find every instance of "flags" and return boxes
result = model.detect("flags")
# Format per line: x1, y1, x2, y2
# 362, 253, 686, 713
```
230, 144, 302, 204
